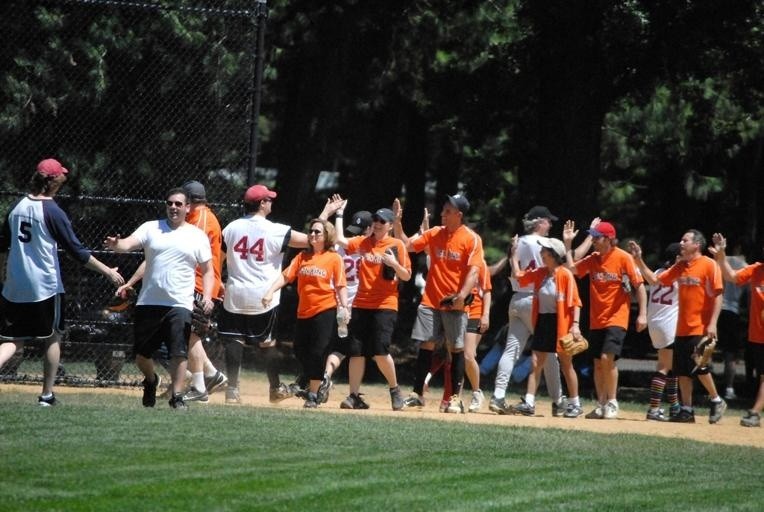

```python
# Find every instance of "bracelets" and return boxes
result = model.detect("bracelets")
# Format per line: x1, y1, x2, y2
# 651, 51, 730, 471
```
333, 214, 343, 218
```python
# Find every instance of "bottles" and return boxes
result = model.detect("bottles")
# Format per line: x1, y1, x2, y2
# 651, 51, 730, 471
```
335, 305, 349, 338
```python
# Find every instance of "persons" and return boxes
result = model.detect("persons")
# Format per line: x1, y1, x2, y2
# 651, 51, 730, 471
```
414, 198, 764, 425
220, 185, 345, 404
100, 187, 214, 411
262, 215, 352, 413
388, 192, 483, 419
114, 179, 228, 402
0, 157, 123, 406
